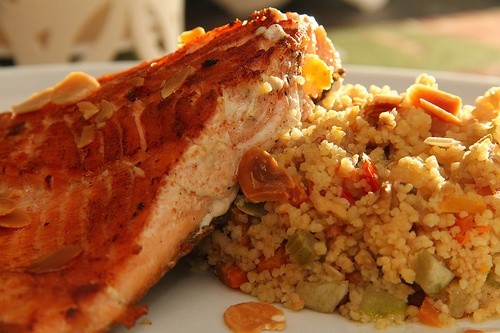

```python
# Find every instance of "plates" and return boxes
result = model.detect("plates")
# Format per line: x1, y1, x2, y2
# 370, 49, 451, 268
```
0, 60, 500, 333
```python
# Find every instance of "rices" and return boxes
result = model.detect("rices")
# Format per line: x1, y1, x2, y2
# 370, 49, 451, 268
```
183, 74, 500, 332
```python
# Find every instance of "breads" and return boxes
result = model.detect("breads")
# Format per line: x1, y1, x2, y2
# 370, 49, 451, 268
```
0, 8, 347, 332
